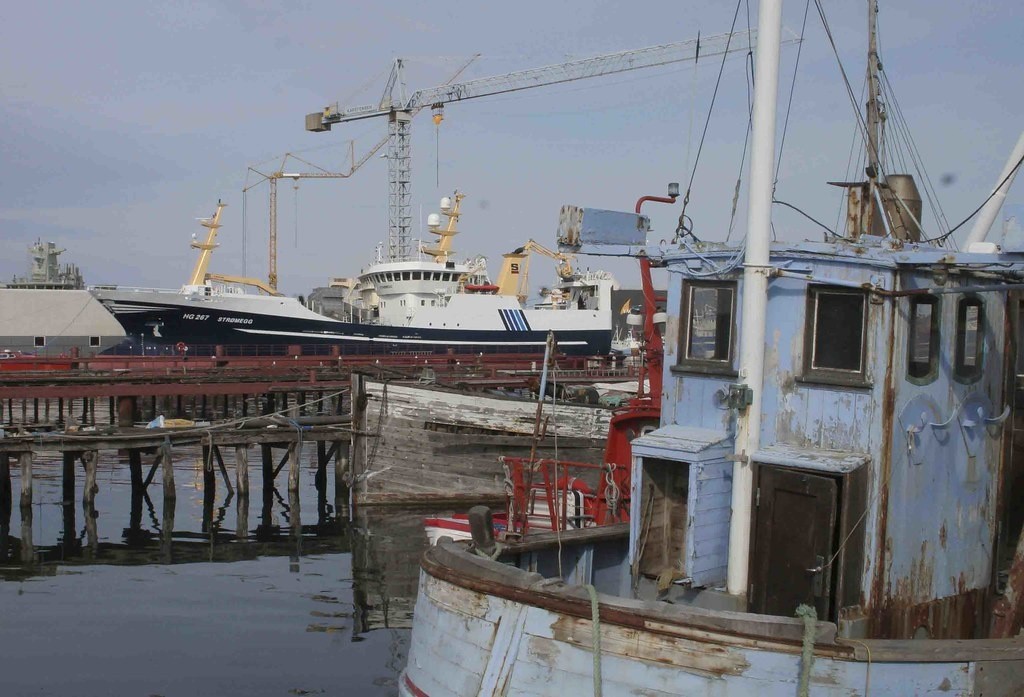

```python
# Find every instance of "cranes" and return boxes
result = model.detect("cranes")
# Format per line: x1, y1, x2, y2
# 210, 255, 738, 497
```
305, 19, 806, 263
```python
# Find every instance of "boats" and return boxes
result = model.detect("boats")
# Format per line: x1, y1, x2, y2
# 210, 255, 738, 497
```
0, 1, 1024, 694
